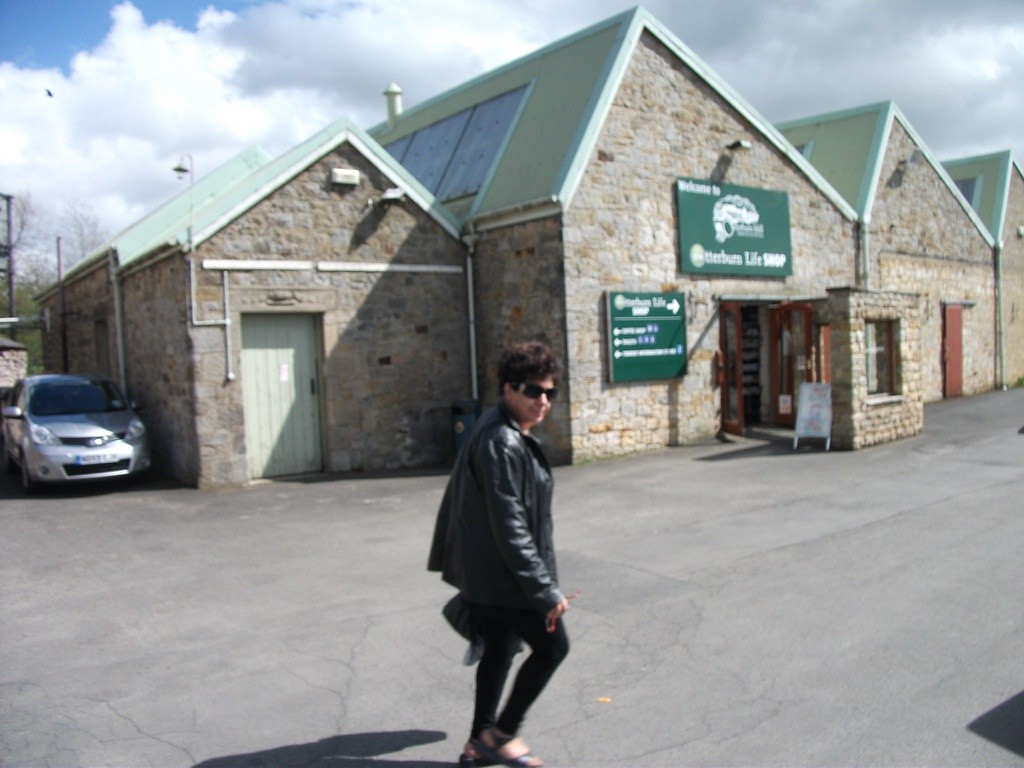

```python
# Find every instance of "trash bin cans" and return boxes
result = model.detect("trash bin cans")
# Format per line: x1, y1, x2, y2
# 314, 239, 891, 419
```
452, 400, 483, 467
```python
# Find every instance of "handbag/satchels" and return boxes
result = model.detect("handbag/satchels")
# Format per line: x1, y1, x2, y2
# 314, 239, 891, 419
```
443, 591, 524, 666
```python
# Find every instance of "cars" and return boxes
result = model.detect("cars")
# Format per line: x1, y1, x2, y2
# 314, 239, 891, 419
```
2, 375, 151, 493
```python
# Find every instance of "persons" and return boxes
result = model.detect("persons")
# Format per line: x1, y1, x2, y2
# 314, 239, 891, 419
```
426, 340, 571, 768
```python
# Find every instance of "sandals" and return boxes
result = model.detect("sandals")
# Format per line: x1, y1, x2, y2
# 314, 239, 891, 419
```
469, 726, 541, 768
459, 742, 496, 768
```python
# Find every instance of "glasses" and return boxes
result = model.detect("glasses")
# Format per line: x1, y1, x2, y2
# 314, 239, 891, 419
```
507, 380, 559, 402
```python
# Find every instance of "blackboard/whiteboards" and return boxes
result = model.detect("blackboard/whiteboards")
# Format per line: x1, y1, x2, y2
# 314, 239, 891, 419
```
793, 381, 832, 438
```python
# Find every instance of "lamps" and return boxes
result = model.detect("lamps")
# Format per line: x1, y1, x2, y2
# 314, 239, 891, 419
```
727, 140, 752, 151
370, 187, 407, 209
172, 152, 194, 245
